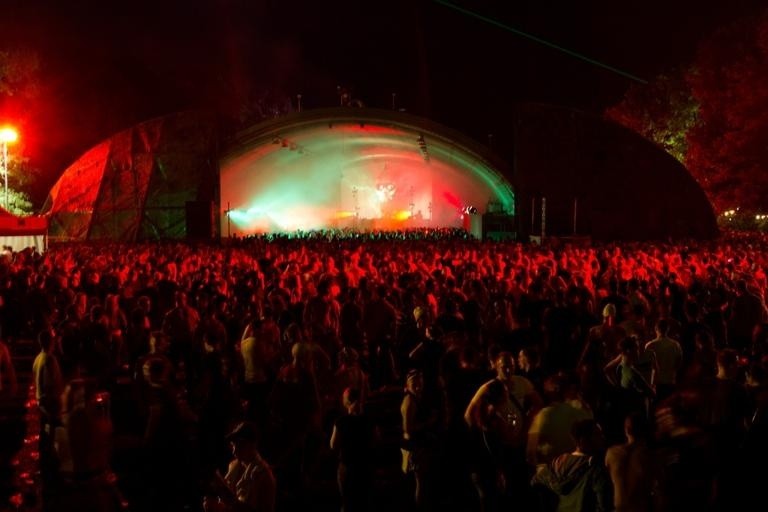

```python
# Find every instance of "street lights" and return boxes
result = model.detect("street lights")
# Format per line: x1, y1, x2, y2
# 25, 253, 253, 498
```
224, 201, 234, 237
0, 128, 16, 211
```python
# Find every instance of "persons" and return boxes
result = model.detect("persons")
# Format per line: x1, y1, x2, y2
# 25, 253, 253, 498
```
1, 219, 768, 512
